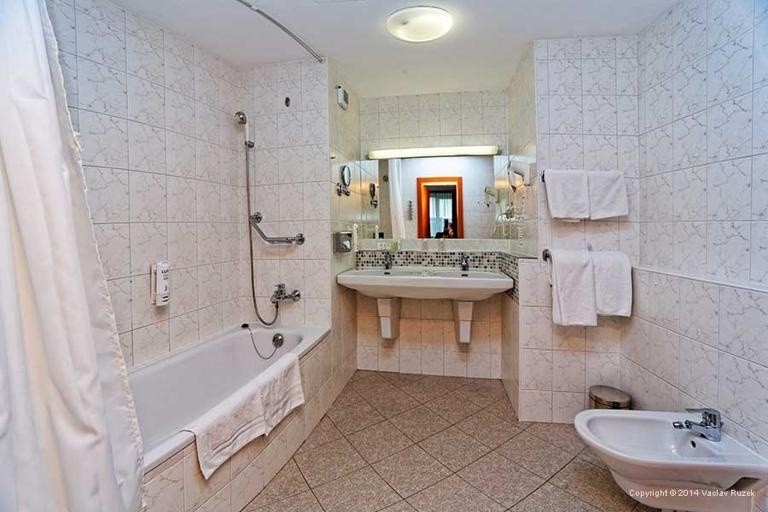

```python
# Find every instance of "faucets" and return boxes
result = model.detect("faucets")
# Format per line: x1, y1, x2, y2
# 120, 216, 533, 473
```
382, 252, 393, 267
462, 255, 470, 271
272, 285, 300, 303
673, 406, 723, 442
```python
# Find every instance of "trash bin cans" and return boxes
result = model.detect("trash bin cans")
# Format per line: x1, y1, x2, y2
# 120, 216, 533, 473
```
589, 384, 631, 409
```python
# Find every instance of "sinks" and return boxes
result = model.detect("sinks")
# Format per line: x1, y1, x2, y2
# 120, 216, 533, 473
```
354, 270, 421, 275
431, 271, 495, 280
574, 409, 768, 512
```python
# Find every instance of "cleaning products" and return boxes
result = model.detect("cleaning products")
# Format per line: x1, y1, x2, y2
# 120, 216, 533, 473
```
336, 231, 354, 252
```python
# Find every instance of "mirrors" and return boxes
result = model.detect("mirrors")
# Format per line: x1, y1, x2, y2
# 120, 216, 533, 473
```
357, 155, 508, 252
336, 165, 351, 196
370, 183, 377, 207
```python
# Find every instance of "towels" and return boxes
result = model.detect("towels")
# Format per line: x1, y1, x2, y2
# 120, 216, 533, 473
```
182, 384, 266, 480
590, 252, 633, 316
588, 170, 630, 220
544, 169, 591, 222
547, 249, 597, 327
252, 353, 305, 437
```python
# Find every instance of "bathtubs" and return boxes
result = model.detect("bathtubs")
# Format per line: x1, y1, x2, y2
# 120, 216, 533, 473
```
130, 325, 331, 473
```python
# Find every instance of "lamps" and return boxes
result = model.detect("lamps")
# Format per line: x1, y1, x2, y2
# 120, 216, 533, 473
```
368, 145, 499, 160
386, 6, 453, 42
